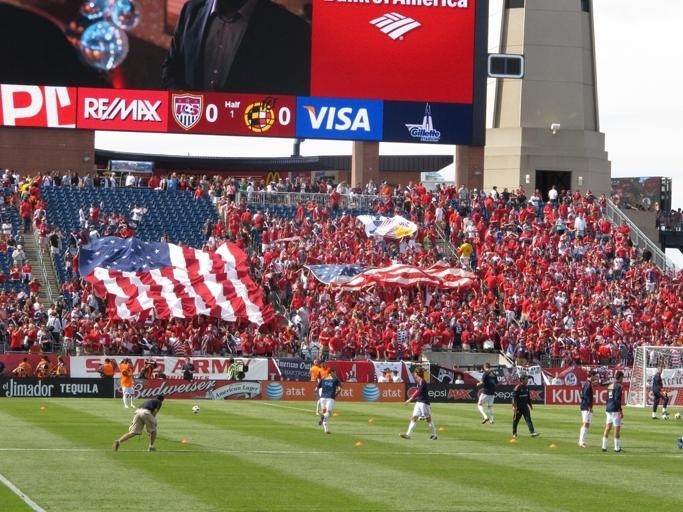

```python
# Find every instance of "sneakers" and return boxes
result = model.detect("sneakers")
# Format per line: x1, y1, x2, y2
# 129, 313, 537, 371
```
399, 417, 542, 441
316, 410, 331, 434
578, 411, 670, 453
113, 403, 157, 452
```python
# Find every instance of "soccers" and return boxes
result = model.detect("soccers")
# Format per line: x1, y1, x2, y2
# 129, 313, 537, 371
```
675, 413, 680, 419
191, 406, 200, 413
662, 414, 670, 421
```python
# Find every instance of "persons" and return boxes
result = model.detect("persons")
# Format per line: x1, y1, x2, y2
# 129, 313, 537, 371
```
108, 393, 164, 451
600, 369, 626, 453
650, 365, 670, 419
119, 361, 138, 408
576, 369, 601, 449
0, 0, 112, 86
473, 361, 499, 426
0, 163, 683, 388
157, 0, 314, 97
398, 367, 438, 441
509, 374, 542, 440
316, 366, 340, 435
